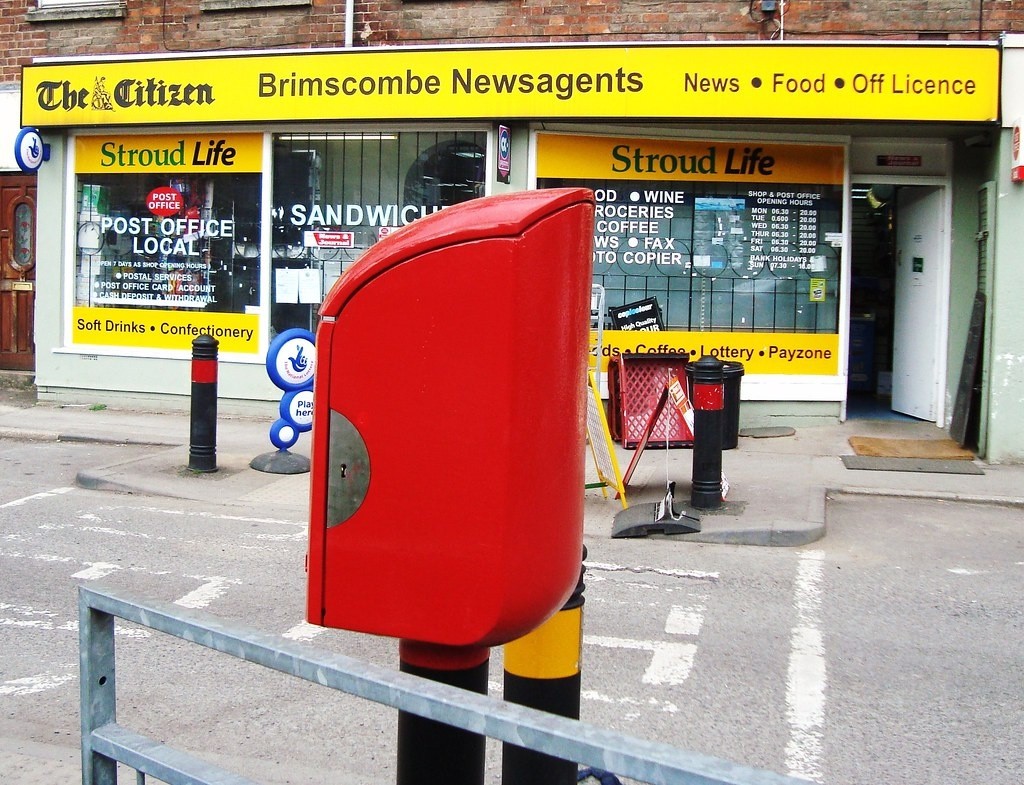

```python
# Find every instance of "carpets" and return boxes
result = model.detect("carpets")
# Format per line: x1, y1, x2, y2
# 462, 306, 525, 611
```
839, 455, 985, 475
848, 436, 974, 460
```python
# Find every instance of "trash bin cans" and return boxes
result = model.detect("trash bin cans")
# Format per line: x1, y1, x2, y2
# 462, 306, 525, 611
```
684, 361, 744, 450
